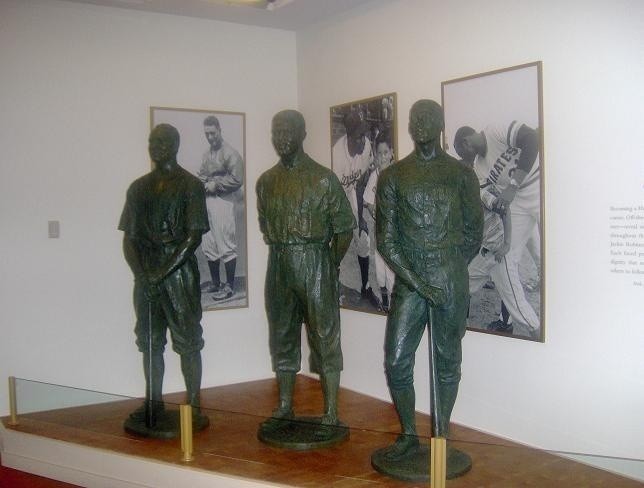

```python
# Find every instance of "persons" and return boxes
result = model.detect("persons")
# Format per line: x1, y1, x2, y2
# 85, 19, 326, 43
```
376, 100, 484, 461
456, 120, 542, 339
255, 109, 358, 437
199, 116, 243, 301
119, 122, 212, 420
332, 109, 396, 313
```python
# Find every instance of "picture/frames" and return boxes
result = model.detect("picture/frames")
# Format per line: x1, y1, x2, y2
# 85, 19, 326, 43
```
328, 91, 399, 317
439, 60, 546, 344
149, 105, 249, 310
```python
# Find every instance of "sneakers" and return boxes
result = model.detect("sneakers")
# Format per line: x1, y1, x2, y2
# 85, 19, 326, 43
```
201, 284, 234, 301
487, 321, 513, 334
361, 287, 388, 312
